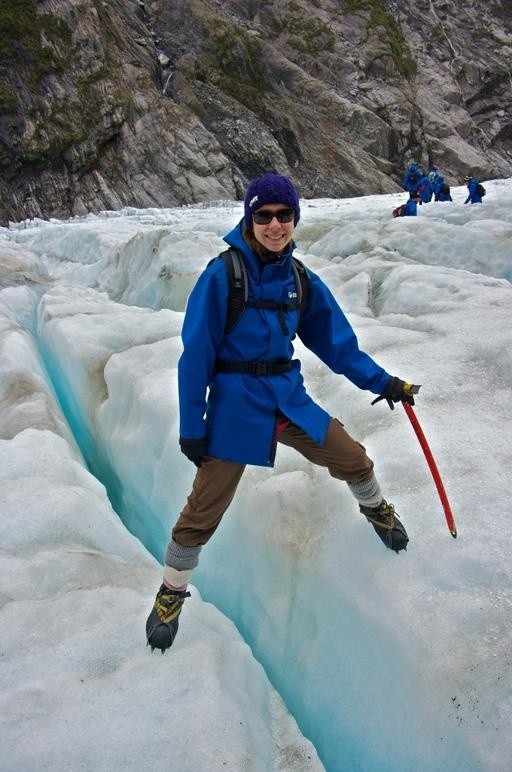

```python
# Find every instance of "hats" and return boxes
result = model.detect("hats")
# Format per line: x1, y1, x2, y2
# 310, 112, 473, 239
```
244, 175, 299, 227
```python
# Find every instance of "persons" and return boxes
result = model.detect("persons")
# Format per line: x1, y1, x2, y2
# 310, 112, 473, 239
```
414, 171, 436, 205
403, 165, 426, 197
146, 175, 415, 648
464, 175, 482, 204
428, 171, 452, 202
406, 191, 421, 216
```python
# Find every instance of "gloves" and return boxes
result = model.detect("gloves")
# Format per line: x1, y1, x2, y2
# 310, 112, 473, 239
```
181, 442, 206, 467
371, 377, 415, 410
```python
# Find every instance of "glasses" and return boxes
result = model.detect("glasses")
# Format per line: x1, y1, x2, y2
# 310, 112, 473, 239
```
251, 209, 295, 225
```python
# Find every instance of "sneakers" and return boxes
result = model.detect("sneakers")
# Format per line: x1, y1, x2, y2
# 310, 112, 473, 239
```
147, 584, 192, 649
361, 499, 409, 549
